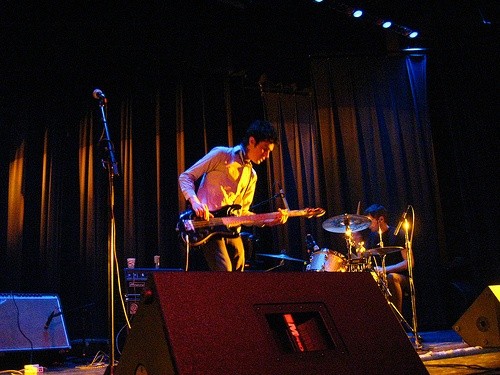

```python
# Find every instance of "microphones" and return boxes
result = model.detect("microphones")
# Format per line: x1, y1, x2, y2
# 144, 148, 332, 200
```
277, 181, 290, 210
44, 310, 55, 329
93, 88, 105, 99
308, 234, 319, 251
394, 206, 410, 235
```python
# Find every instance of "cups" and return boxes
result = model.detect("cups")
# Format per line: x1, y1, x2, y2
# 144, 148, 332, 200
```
24, 364, 38, 375
127, 258, 135, 269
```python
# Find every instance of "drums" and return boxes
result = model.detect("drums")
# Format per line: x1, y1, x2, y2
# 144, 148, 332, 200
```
306, 249, 349, 272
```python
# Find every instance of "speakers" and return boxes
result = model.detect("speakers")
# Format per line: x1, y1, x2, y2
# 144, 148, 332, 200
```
0, 293, 72, 371
452, 284, 500, 348
115, 270, 430, 375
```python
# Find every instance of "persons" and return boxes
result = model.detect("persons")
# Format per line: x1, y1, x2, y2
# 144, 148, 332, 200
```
364, 206, 415, 314
178, 119, 289, 272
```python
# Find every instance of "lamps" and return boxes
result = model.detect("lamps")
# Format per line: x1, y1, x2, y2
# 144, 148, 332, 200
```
349, 1, 419, 39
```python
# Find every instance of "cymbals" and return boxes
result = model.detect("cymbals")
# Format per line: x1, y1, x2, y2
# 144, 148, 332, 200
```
366, 247, 404, 255
256, 253, 309, 262
322, 214, 372, 233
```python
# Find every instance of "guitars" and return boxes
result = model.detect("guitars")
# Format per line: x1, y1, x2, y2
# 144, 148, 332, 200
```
178, 204, 326, 246
